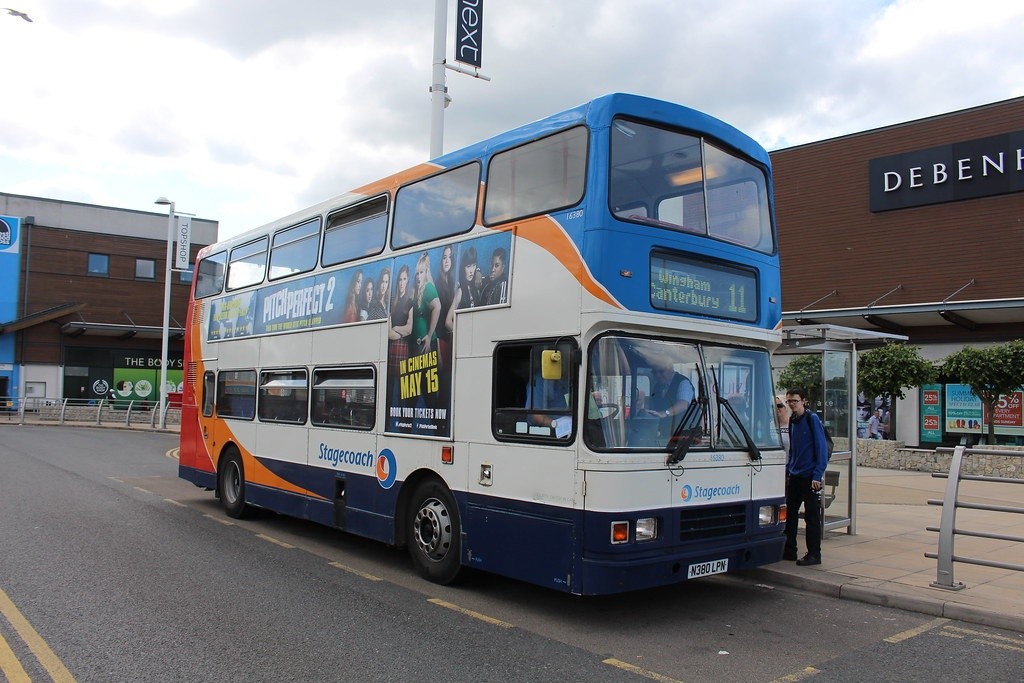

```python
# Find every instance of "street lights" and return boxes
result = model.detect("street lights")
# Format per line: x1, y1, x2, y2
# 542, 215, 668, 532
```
151, 193, 177, 429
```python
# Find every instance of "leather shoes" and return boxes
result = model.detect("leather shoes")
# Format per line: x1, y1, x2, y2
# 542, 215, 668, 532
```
796, 553, 822, 566
782, 551, 797, 561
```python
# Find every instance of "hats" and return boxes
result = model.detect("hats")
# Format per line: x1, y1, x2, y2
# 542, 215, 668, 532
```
109, 389, 115, 392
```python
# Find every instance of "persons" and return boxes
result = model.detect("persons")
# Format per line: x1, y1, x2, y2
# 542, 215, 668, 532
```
857, 406, 870, 422
771, 387, 833, 566
343, 244, 506, 438
725, 394, 751, 437
650, 355, 702, 441
527, 373, 572, 433
868, 410, 890, 440
856, 427, 867, 439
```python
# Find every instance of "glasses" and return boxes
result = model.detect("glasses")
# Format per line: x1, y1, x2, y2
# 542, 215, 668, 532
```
777, 403, 785, 408
650, 367, 667, 375
786, 399, 803, 404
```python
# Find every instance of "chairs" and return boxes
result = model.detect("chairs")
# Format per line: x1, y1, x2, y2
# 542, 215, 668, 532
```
217, 393, 374, 427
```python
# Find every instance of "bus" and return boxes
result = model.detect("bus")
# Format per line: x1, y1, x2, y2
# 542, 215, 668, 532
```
175, 92, 790, 603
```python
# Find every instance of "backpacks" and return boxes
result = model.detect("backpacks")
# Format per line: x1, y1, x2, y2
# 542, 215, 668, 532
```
806, 408, 835, 463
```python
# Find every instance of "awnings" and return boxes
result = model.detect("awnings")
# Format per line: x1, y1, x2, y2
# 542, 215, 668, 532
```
782, 297, 1024, 329
60, 321, 184, 339
